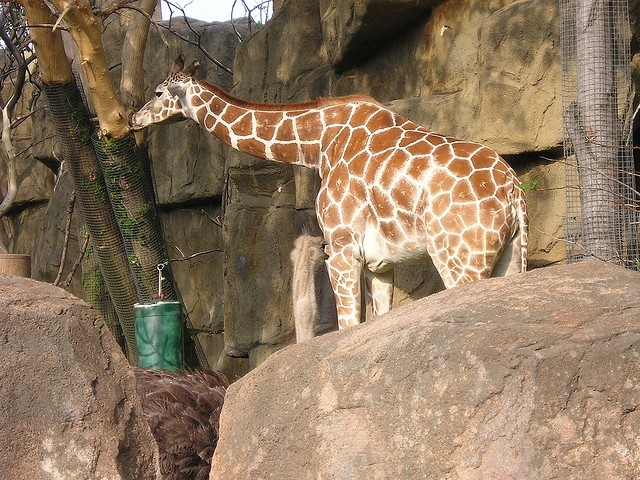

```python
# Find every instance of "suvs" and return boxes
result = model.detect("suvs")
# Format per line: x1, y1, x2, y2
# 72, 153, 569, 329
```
133, 301, 183, 375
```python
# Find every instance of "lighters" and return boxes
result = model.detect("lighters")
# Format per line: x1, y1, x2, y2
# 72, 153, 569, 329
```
126, 52, 531, 330
